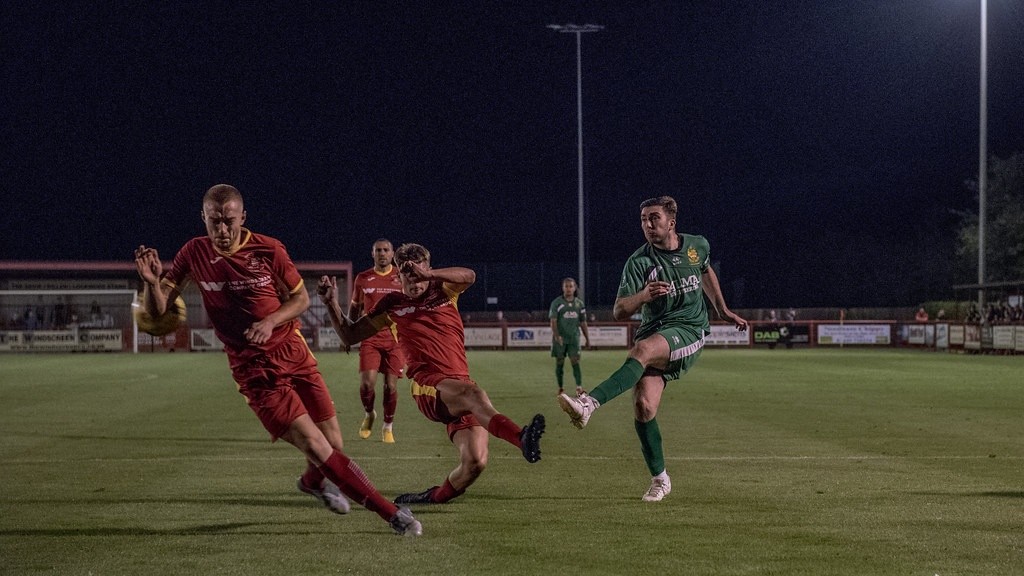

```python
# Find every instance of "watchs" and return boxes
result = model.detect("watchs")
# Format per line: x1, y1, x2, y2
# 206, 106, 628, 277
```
557, 196, 748, 502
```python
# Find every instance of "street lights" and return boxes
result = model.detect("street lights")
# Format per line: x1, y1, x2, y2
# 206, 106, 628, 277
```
549, 19, 607, 313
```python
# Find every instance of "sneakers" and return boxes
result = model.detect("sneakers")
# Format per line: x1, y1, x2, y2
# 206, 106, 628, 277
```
557, 388, 566, 398
642, 475, 671, 503
391, 486, 439, 505
575, 387, 586, 397
560, 392, 597, 430
520, 413, 547, 463
389, 503, 423, 537
358, 412, 376, 439
296, 475, 350, 515
380, 421, 396, 443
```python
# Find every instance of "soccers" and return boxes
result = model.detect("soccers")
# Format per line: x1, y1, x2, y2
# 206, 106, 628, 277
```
131, 293, 187, 336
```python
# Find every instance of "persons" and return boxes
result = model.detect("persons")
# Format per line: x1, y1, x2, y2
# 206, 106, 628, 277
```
764, 309, 779, 349
935, 308, 945, 321
914, 308, 929, 323
316, 244, 545, 503
8, 300, 113, 330
341, 237, 405, 443
782, 311, 796, 349
549, 277, 591, 397
133, 182, 422, 539
962, 301, 1024, 356
